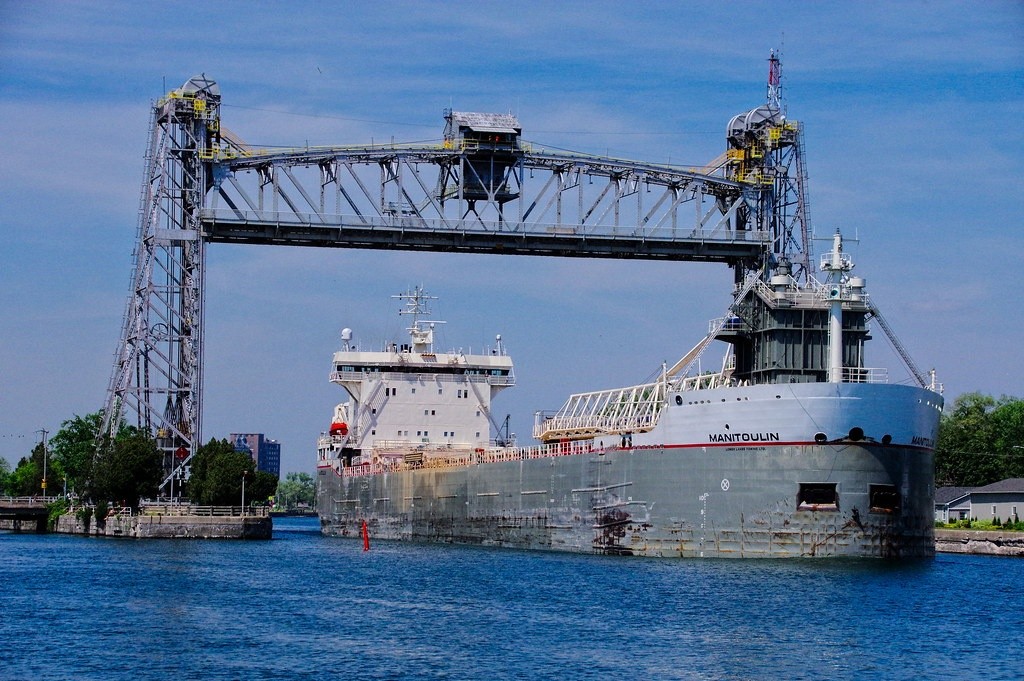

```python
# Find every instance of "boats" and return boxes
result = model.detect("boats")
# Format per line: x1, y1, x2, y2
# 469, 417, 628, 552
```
329, 421, 348, 437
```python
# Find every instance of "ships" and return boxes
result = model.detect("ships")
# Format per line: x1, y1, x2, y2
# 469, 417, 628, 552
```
316, 225, 945, 562
